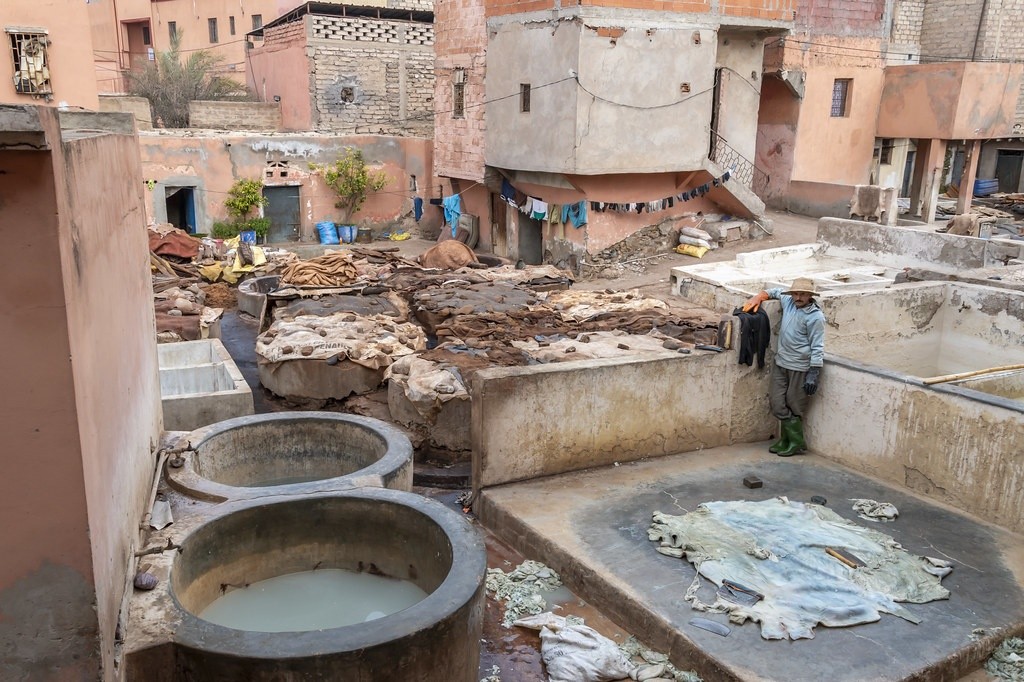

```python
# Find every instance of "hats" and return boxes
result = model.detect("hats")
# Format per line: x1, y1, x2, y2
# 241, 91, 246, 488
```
780, 278, 821, 296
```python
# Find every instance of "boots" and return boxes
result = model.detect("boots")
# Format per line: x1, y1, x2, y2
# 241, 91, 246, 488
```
769, 421, 789, 453
777, 417, 807, 456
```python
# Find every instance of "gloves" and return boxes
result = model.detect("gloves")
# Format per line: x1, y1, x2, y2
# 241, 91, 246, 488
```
742, 291, 769, 313
801, 366, 821, 395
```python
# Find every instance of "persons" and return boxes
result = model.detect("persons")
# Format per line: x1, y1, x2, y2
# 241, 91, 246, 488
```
742, 276, 826, 457
